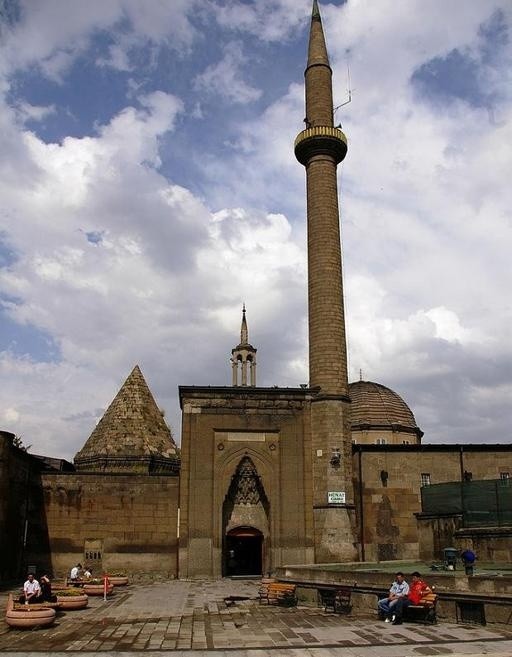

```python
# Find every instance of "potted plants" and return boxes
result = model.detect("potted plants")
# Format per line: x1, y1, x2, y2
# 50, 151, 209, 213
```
5, 567, 128, 628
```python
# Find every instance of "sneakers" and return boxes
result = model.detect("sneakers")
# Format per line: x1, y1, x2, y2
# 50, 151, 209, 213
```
384, 614, 402, 624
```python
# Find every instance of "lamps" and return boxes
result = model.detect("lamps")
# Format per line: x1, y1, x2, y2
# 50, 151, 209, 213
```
330, 447, 341, 465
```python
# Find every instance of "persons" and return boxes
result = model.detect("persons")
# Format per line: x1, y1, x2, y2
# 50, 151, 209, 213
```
19, 572, 44, 604
461, 546, 475, 574
34, 574, 52, 603
384, 570, 432, 625
83, 567, 93, 577
70, 562, 82, 586
376, 571, 409, 622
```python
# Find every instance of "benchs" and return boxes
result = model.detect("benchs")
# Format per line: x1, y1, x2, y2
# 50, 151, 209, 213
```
258, 583, 437, 625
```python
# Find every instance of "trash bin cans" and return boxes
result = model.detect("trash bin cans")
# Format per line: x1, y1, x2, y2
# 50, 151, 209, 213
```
443, 547, 459, 571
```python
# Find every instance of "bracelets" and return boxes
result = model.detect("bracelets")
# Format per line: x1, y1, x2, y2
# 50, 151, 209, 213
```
420, 590, 422, 595
